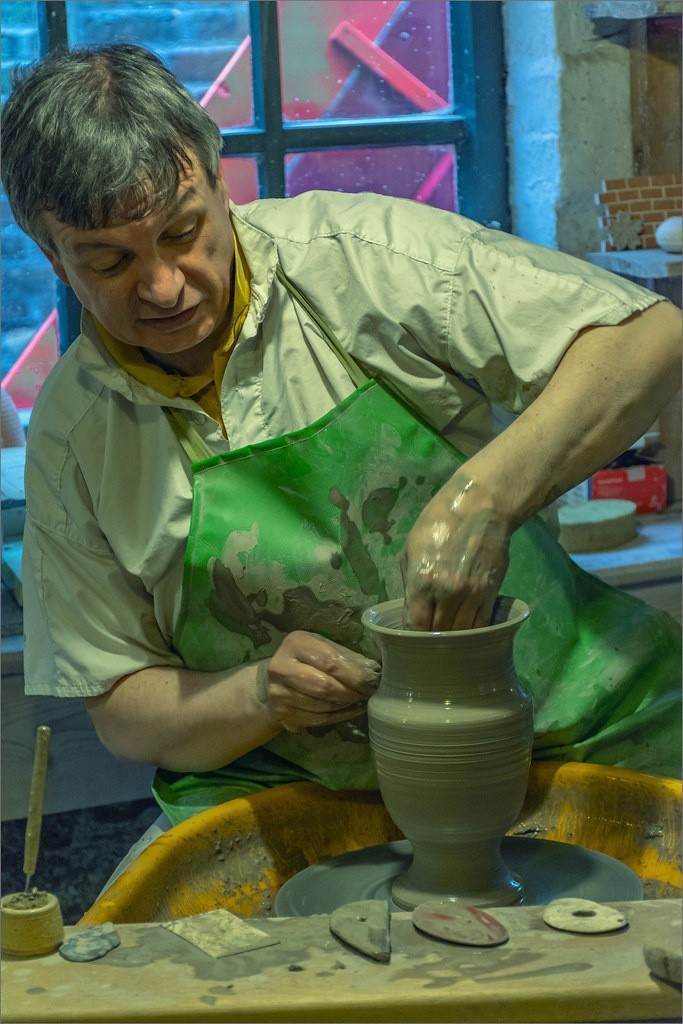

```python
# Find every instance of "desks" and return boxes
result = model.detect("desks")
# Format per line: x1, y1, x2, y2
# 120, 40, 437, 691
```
589, 247, 683, 293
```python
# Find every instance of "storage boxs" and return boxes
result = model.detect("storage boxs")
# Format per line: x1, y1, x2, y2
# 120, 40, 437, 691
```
588, 451, 668, 516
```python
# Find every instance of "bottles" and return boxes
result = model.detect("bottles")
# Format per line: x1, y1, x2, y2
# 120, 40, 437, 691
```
358, 595, 536, 913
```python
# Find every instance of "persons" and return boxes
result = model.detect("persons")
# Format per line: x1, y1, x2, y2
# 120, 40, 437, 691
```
0, 38, 683, 926
